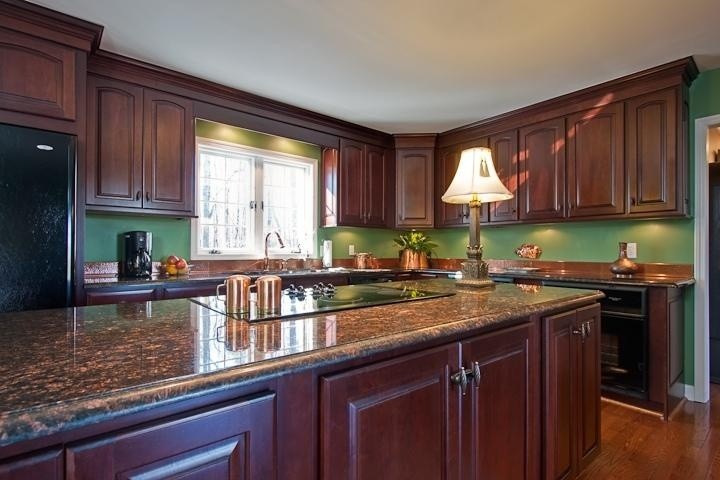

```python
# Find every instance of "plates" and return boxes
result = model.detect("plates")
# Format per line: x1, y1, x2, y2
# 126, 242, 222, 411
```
506, 266, 542, 272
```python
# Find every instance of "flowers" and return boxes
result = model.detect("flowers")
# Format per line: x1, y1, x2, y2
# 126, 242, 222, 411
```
390, 230, 444, 264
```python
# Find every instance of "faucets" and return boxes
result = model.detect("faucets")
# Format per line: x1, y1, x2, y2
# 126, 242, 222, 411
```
263, 230, 285, 271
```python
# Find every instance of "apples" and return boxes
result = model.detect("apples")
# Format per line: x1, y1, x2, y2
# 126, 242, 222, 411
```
160, 255, 188, 274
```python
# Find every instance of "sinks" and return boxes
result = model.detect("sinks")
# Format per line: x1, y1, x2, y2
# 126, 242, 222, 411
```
219, 270, 301, 276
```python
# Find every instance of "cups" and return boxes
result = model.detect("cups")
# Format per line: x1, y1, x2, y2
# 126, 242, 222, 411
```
216, 274, 251, 314
246, 275, 284, 315
247, 314, 282, 353
217, 316, 251, 352
353, 253, 370, 268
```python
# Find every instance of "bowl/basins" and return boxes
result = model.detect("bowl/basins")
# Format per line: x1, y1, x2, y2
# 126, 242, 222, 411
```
156, 265, 195, 275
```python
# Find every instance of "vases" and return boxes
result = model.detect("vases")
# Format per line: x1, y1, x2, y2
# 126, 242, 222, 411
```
399, 249, 429, 270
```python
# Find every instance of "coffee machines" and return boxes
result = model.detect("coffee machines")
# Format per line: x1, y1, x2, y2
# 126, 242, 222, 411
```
121, 230, 153, 277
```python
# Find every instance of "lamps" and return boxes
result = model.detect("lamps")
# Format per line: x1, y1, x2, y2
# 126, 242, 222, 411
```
443, 146, 515, 287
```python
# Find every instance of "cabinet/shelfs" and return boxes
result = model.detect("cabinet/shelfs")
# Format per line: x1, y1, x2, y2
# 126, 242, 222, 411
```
540, 297, 603, 480
395, 130, 438, 230
0, 360, 301, 480
625, 54, 700, 220
321, 113, 395, 230
302, 310, 543, 480
519, 72, 625, 226
85, 283, 163, 306
281, 273, 349, 287
85, 43, 197, 221
163, 282, 226, 302
350, 272, 409, 285
435, 117, 488, 230
0, 0, 105, 135
488, 108, 519, 226
